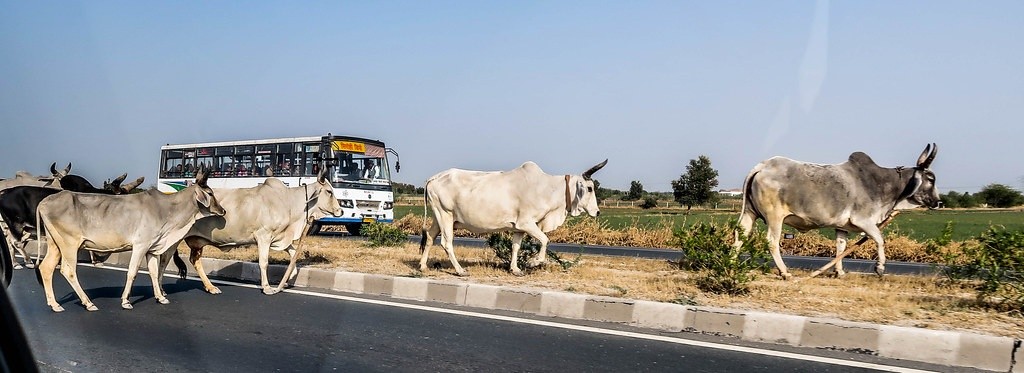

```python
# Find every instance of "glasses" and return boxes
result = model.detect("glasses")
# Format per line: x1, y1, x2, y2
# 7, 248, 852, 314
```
286, 165, 289, 166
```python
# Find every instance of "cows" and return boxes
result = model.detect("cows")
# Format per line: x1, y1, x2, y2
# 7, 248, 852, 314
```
729, 142, 946, 282
419, 159, 611, 277
0, 161, 146, 270
182, 160, 345, 296
33, 162, 226, 310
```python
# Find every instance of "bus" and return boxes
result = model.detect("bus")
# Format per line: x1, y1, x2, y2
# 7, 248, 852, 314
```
157, 133, 400, 235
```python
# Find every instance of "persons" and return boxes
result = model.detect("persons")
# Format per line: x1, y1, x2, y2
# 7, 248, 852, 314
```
164, 161, 363, 181
363, 160, 380, 182
